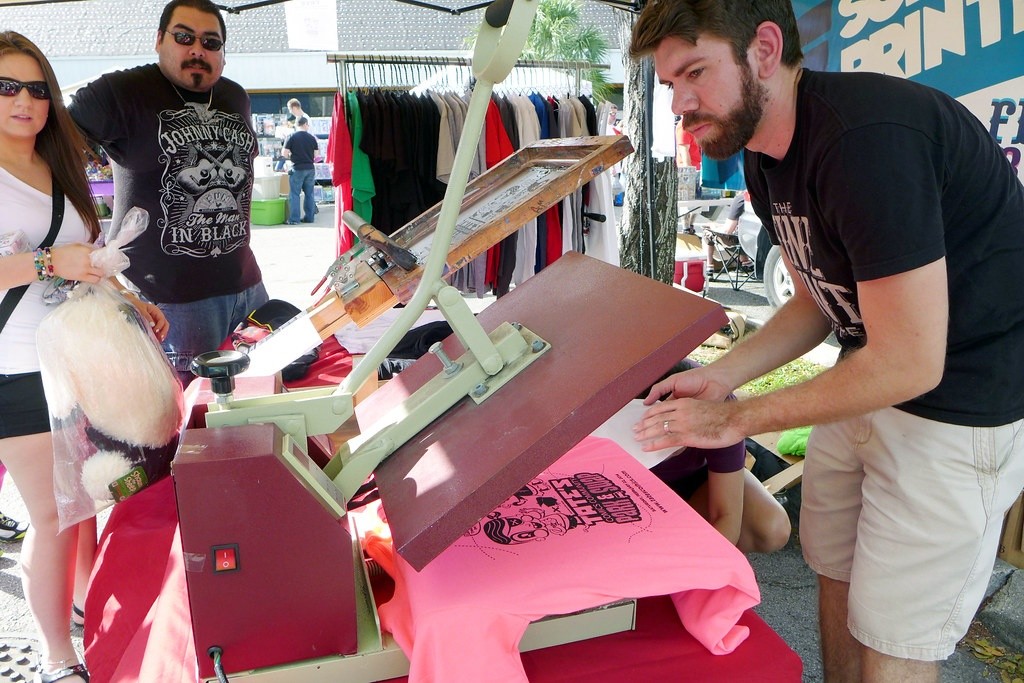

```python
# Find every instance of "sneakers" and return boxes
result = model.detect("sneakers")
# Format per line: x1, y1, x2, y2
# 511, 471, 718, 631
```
0, 510, 34, 544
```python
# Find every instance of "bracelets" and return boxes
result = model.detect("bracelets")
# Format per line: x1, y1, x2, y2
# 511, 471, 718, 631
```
119, 288, 137, 297
32, 246, 54, 282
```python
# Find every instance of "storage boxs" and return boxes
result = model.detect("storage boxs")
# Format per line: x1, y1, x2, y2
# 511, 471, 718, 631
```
251, 198, 287, 225
677, 166, 696, 201
252, 172, 282, 200
280, 193, 306, 221
280, 174, 290, 194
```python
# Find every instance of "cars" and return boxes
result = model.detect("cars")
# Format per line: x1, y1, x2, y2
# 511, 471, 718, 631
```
675, 184, 797, 314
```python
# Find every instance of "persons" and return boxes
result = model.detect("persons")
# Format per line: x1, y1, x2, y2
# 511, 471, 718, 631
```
0, 28, 171, 683
66, 0, 270, 391
274, 97, 321, 225
627, 1, 1023, 683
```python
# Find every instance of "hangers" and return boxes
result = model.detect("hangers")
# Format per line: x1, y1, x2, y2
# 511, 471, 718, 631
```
398, 55, 406, 91
389, 55, 396, 96
393, 55, 402, 96
334, 53, 341, 96
416, 56, 429, 93
362, 55, 370, 91
352, 54, 361, 93
410, 56, 418, 88
423, 56, 583, 111
369, 54, 374, 93
403, 56, 412, 90
581, 60, 600, 103
372, 55, 379, 90
345, 54, 354, 91
590, 60, 618, 109
383, 55, 392, 93
378, 55, 387, 92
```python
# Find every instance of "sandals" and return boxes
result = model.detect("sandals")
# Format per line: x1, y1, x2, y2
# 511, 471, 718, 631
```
35, 652, 91, 683
72, 600, 85, 632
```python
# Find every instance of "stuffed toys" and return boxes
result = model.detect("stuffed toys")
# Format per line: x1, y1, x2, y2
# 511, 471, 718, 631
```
36, 280, 185, 504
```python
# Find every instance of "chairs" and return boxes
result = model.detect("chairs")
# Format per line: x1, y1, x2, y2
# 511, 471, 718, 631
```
701, 225, 758, 292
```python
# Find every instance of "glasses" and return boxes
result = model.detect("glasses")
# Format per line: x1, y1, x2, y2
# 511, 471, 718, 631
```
0, 77, 51, 100
163, 26, 224, 52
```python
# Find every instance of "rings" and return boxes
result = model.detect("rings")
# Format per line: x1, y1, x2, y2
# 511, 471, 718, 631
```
663, 420, 672, 434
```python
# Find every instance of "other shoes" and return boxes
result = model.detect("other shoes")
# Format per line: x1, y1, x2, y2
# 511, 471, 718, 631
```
706, 270, 714, 282
284, 219, 297, 225
299, 218, 312, 222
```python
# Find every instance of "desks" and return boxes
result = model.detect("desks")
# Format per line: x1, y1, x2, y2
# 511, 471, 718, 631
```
83, 323, 805, 683
678, 198, 733, 218
